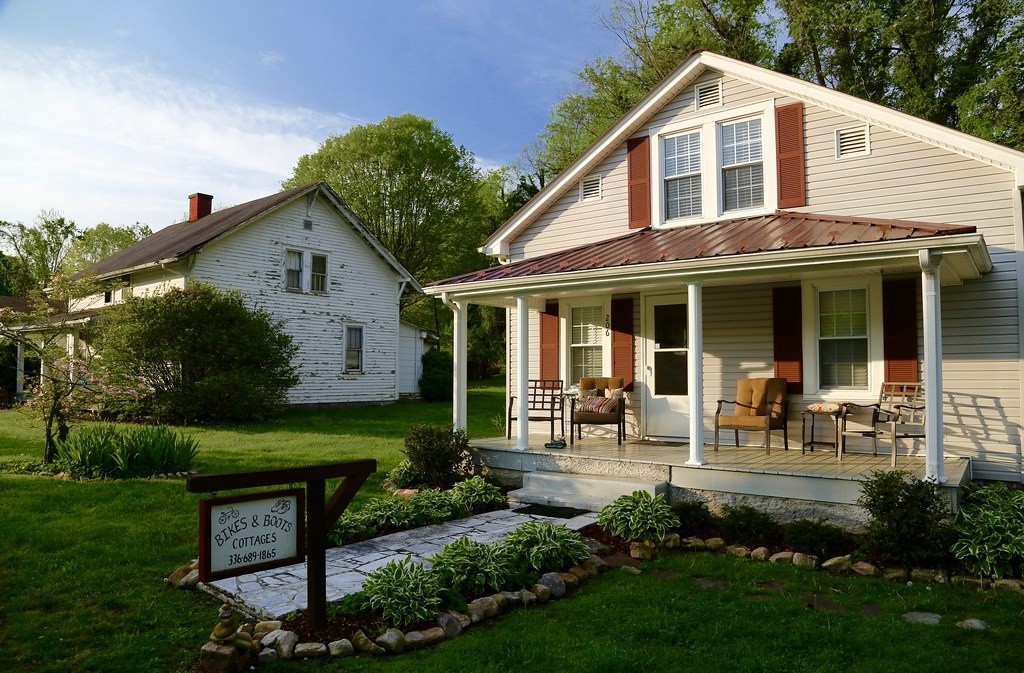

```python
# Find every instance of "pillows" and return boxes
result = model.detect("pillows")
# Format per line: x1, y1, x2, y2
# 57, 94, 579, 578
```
604, 387, 623, 412
807, 402, 849, 415
579, 394, 619, 412
578, 388, 598, 410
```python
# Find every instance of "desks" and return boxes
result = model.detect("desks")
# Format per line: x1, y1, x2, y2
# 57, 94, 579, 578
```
801, 408, 853, 458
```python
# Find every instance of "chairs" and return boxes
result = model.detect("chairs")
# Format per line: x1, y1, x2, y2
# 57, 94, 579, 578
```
569, 376, 627, 447
505, 379, 566, 441
841, 383, 927, 467
714, 378, 790, 455
